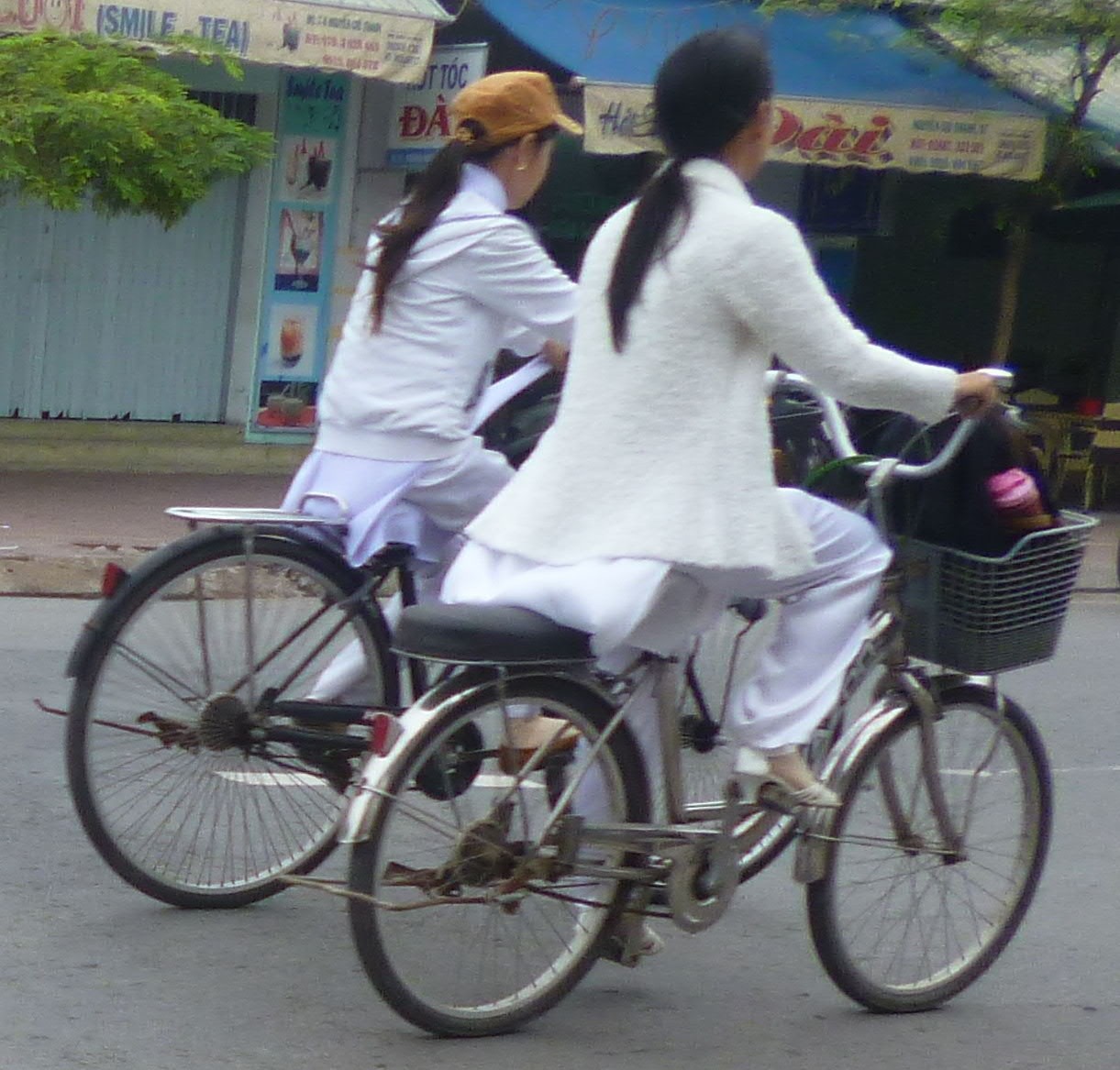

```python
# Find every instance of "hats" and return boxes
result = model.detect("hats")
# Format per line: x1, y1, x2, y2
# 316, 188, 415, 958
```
450, 71, 585, 151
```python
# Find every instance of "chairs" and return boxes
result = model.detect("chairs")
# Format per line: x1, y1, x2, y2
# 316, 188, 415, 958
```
1013, 388, 1120, 513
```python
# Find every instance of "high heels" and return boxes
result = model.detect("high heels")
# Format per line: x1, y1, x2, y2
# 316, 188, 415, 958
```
578, 897, 663, 957
733, 742, 840, 808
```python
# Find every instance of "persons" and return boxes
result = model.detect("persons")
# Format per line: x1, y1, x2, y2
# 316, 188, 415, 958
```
279, 65, 579, 776
441, 28, 1001, 956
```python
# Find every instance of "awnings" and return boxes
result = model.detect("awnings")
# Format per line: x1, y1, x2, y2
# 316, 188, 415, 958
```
482, 0, 1049, 180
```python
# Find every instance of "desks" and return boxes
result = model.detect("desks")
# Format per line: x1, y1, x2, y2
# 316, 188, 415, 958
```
1035, 411, 1100, 501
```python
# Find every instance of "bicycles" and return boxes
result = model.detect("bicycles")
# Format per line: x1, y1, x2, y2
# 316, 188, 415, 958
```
275, 366, 1105, 1042
31, 350, 850, 910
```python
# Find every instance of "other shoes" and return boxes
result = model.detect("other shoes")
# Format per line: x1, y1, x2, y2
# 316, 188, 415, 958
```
498, 732, 584, 773
287, 697, 356, 785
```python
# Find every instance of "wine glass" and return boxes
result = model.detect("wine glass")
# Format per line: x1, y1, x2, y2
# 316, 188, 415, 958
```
292, 235, 313, 291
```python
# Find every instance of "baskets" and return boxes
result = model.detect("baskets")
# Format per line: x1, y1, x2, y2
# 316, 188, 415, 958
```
856, 498, 1101, 675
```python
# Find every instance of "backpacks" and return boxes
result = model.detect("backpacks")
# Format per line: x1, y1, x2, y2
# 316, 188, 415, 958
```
866, 406, 1064, 559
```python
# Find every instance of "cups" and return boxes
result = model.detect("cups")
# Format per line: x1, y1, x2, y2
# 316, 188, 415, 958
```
283, 400, 302, 426
314, 161, 331, 189
267, 397, 284, 420
281, 332, 304, 368
286, 159, 298, 185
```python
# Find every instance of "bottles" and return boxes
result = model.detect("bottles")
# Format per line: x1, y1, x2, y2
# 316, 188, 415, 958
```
986, 466, 1049, 539
